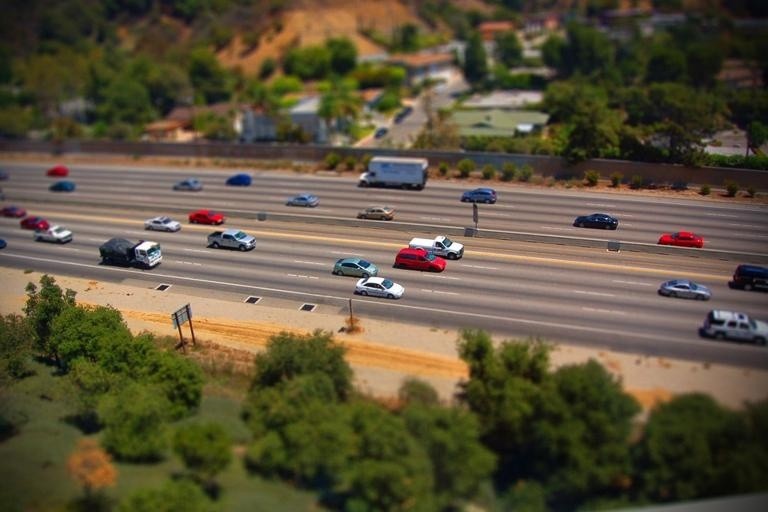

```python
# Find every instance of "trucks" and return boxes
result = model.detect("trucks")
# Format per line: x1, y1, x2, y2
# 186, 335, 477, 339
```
99, 237, 163, 270
359, 156, 429, 191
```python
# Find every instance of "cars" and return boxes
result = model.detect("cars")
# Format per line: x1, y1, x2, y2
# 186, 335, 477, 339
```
391, 104, 415, 123
288, 193, 321, 208
226, 173, 252, 186
173, 177, 203, 191
144, 216, 182, 232
372, 127, 389, 138
659, 231, 704, 248
358, 206, 397, 221
334, 257, 378, 279
1, 165, 76, 250
188, 209, 225, 225
355, 275, 405, 299
660, 279, 712, 300
575, 213, 618, 231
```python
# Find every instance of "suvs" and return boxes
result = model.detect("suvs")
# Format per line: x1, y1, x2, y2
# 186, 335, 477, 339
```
395, 248, 446, 272
704, 310, 768, 345
734, 265, 768, 291
462, 187, 497, 203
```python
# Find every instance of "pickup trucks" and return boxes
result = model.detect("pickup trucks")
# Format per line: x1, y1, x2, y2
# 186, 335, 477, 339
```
409, 235, 465, 259
207, 228, 257, 252
